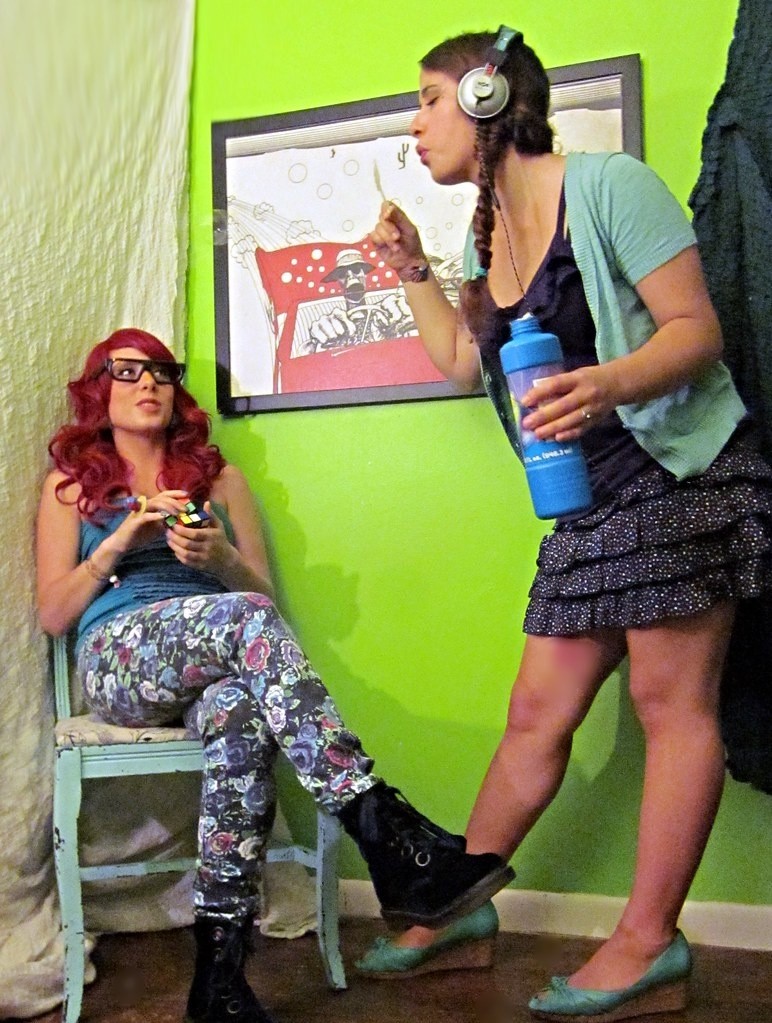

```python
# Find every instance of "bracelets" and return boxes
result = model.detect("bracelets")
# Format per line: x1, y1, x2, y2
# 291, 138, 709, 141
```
397, 260, 431, 284
84, 554, 123, 590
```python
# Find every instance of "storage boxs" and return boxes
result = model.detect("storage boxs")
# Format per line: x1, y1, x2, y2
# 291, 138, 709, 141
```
498, 311, 592, 524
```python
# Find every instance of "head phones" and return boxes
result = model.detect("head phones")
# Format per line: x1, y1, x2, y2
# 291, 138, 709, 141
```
456, 25, 524, 119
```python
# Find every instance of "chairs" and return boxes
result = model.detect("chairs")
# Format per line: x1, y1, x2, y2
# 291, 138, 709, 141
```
52, 633, 351, 1022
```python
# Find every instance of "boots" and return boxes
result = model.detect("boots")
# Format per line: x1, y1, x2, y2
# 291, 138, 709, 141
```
340, 780, 514, 932
186, 918, 267, 1021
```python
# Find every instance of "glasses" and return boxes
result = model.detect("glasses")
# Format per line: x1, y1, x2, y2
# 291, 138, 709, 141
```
87, 357, 186, 386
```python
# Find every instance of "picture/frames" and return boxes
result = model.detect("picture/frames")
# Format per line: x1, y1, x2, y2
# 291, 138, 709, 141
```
211, 52, 643, 416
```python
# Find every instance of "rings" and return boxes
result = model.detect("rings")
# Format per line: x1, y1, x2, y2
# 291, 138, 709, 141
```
580, 407, 592, 419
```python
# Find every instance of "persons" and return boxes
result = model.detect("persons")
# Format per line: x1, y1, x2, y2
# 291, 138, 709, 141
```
32, 324, 516, 1022
346, 25, 772, 1021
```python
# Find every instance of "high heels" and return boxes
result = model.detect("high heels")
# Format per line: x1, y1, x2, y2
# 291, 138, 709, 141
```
528, 928, 694, 1022
354, 899, 500, 982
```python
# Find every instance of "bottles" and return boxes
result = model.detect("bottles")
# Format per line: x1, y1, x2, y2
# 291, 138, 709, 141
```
499, 314, 594, 526
